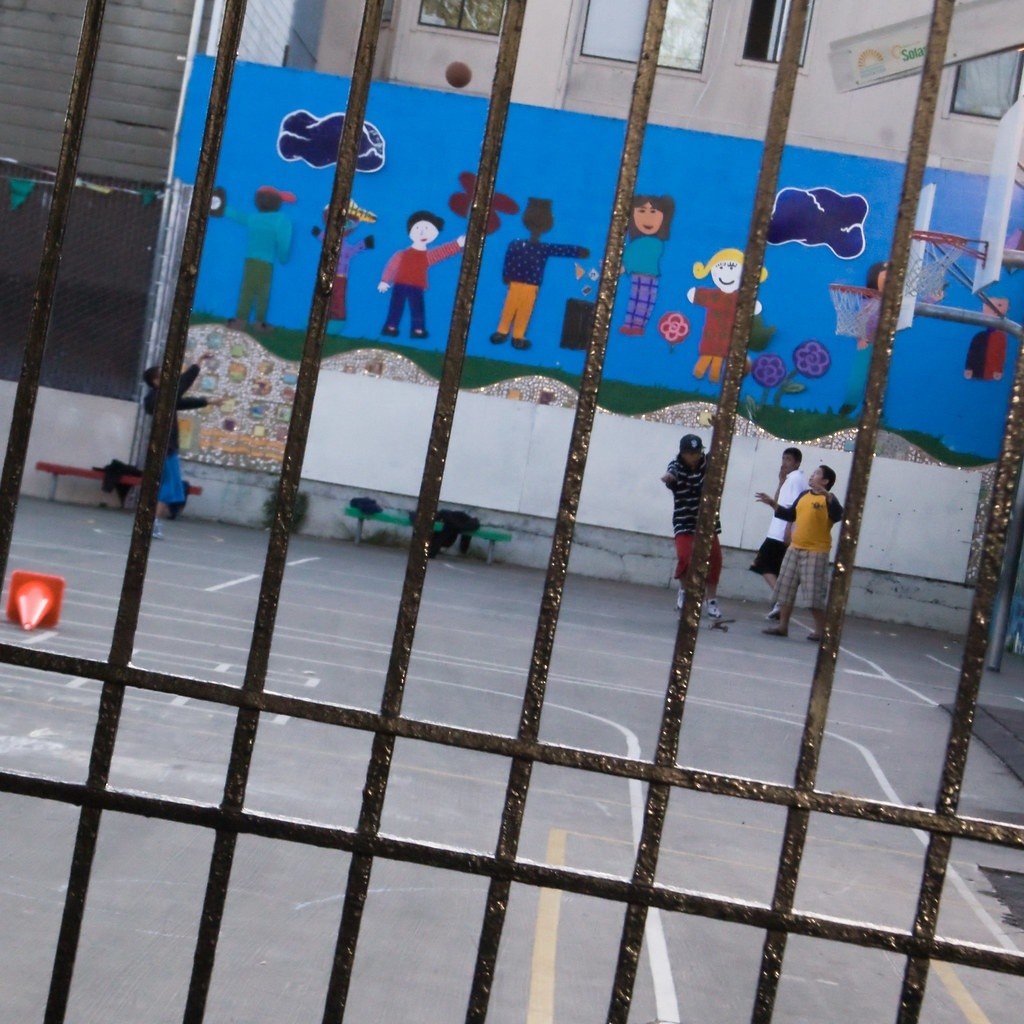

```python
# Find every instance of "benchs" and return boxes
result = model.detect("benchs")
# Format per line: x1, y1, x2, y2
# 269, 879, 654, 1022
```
35, 461, 202, 520
344, 506, 513, 566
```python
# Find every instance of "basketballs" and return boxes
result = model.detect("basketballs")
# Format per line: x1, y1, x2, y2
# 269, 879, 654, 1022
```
445, 59, 473, 89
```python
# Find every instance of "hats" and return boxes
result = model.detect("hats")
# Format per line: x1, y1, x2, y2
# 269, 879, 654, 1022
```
680, 434, 704, 453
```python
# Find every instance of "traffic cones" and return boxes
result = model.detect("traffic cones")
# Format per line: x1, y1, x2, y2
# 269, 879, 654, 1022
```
8, 571, 65, 633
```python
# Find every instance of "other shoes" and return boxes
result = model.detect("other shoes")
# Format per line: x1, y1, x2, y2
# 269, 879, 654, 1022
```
762, 626, 787, 637
767, 601, 781, 619
807, 632, 820, 642
677, 587, 687, 609
152, 517, 162, 538
706, 598, 722, 621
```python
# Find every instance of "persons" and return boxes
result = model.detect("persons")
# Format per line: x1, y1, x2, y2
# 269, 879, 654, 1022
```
749, 448, 808, 621
142, 348, 232, 541
660, 434, 725, 618
754, 465, 844, 641
